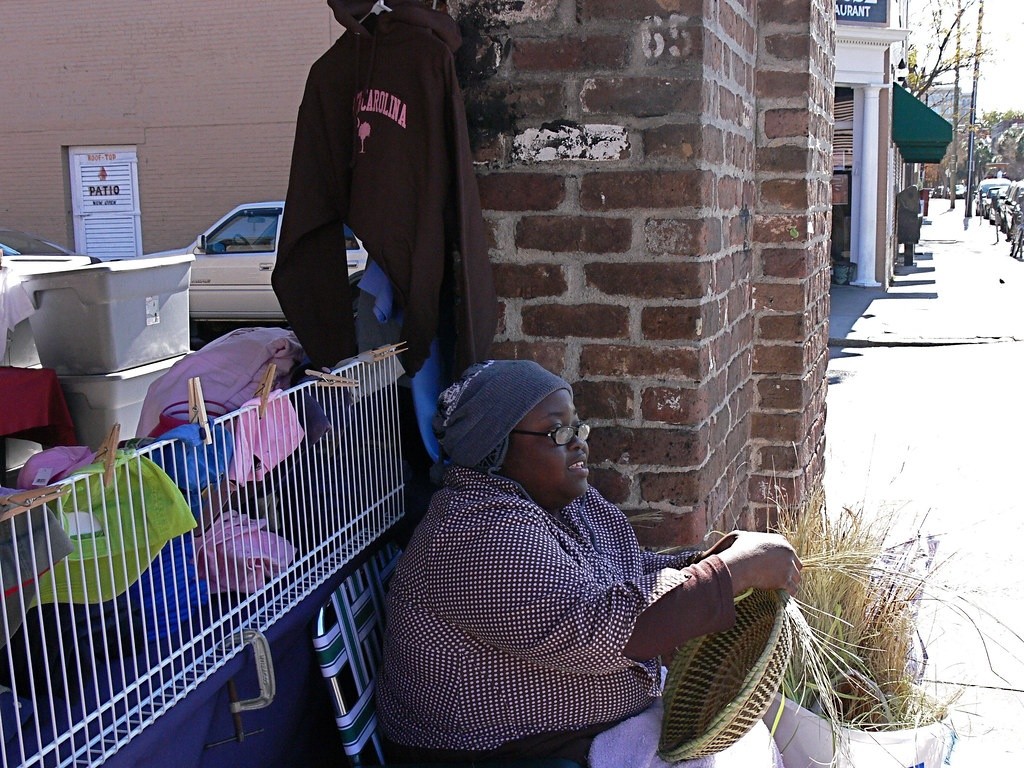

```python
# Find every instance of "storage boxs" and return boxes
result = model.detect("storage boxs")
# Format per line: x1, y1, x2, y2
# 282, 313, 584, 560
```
59, 350, 195, 454
25, 253, 196, 376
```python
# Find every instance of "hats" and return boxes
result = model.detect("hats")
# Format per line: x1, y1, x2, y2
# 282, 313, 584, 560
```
435, 361, 572, 468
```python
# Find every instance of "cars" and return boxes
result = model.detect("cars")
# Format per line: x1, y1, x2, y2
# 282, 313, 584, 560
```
974, 178, 1024, 241
0, 225, 83, 260
948, 184, 966, 198
111, 201, 369, 326
933, 186, 944, 197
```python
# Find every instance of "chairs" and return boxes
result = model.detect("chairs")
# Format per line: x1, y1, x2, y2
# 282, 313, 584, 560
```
311, 538, 404, 768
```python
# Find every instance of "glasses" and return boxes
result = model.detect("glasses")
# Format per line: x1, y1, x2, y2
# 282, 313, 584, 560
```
510, 420, 591, 446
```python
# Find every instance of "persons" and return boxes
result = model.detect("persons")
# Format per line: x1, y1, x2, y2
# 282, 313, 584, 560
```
381, 361, 802, 768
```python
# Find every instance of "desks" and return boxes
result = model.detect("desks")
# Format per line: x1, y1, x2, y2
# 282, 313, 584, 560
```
0, 366, 77, 488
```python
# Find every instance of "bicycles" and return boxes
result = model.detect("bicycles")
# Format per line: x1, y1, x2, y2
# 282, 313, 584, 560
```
1003, 209, 1024, 258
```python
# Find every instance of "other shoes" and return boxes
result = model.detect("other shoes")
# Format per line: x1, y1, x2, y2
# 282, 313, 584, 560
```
1006, 237, 1011, 242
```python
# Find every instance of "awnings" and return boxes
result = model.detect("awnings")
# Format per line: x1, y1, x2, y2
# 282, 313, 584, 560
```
893, 81, 953, 164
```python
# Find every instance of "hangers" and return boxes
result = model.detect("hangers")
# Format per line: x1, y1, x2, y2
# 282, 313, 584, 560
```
357, 0, 392, 25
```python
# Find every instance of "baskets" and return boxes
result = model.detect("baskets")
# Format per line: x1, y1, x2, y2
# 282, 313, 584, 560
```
655, 582, 806, 761
